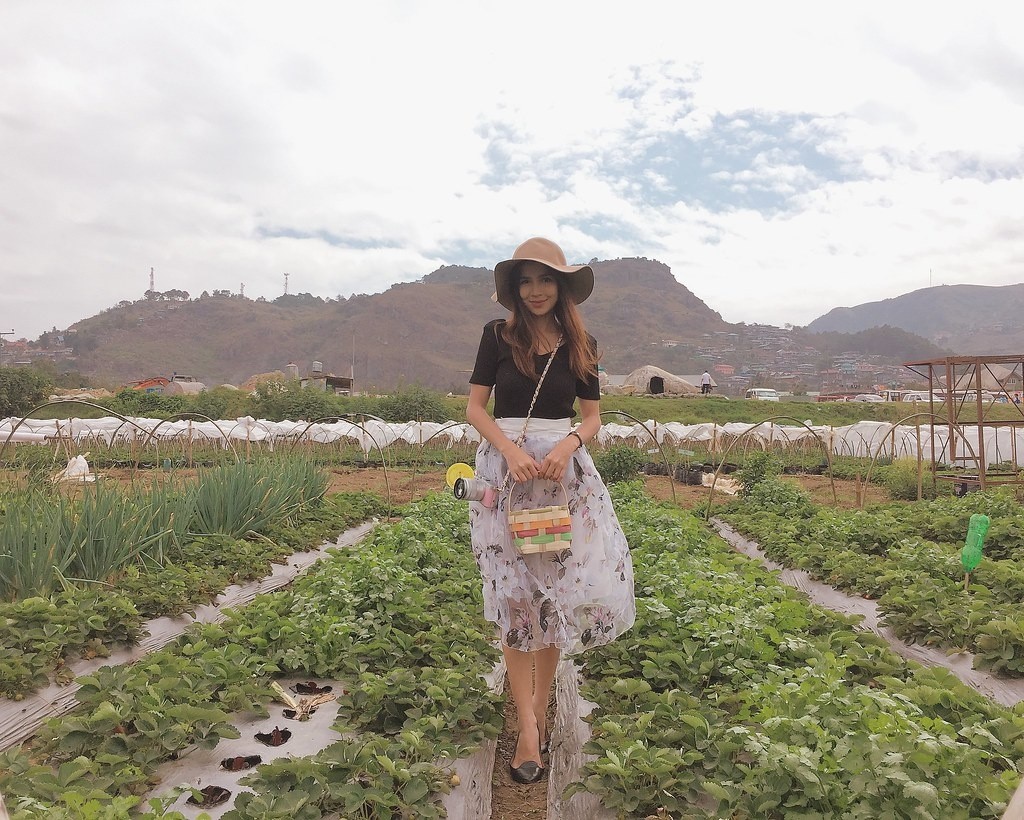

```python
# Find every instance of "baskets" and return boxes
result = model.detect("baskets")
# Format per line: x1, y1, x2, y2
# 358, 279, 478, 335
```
508, 471, 572, 555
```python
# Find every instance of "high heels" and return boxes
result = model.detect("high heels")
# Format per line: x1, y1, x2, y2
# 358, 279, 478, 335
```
537, 722, 551, 753
509, 726, 545, 784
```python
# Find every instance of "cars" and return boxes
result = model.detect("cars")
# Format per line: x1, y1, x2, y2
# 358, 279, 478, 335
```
962, 390, 997, 403
903, 392, 944, 403
854, 394, 885, 402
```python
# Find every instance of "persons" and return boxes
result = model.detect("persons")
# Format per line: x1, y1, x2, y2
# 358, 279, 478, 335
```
1014, 393, 1021, 405
701, 370, 711, 394
467, 234, 637, 784
1000, 395, 1008, 404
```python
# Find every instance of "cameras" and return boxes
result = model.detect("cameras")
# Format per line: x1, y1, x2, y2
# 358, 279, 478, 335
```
454, 477, 500, 508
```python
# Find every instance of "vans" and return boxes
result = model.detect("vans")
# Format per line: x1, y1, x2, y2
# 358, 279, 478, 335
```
745, 387, 781, 402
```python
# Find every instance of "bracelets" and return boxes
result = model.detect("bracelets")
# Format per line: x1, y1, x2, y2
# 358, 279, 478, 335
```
565, 431, 583, 452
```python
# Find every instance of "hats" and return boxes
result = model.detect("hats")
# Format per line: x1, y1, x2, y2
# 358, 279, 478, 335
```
490, 237, 594, 313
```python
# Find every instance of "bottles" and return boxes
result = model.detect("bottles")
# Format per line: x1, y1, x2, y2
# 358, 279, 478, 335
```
163, 458, 171, 473
960, 513, 990, 573
